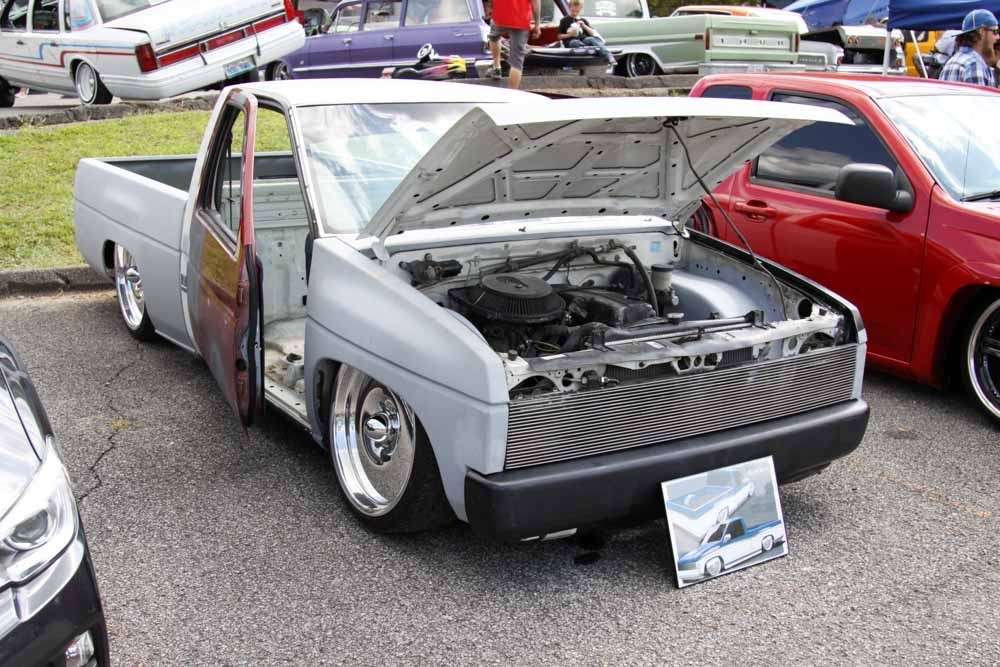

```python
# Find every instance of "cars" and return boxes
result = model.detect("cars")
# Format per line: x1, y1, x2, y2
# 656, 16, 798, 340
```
669, 4, 844, 73
264, 0, 501, 86
0, 1, 306, 109
0, 338, 112, 667
784, 0, 952, 80
659, 67, 1000, 427
483, 0, 619, 79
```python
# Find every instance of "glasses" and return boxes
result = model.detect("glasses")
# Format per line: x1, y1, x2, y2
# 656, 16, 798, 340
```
981, 28, 999, 35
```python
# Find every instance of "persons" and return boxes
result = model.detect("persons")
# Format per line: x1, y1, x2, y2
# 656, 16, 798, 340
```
557, 0, 618, 65
484, 0, 542, 91
938, 10, 1000, 87
482, 0, 495, 26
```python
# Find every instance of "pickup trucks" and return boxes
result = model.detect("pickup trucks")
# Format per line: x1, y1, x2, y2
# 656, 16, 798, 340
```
70, 75, 881, 537
678, 517, 785, 584
564, 1, 806, 80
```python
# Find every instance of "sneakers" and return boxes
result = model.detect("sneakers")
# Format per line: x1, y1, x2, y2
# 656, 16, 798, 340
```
484, 67, 501, 78
600, 53, 618, 65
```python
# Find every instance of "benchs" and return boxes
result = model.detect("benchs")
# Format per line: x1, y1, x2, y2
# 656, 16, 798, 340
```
498, 25, 622, 67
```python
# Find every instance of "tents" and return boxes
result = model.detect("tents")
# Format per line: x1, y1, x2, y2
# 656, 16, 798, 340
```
882, 0, 1000, 74
783, 1, 891, 33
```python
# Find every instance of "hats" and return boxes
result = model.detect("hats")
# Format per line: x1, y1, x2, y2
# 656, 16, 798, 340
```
951, 9, 998, 36
